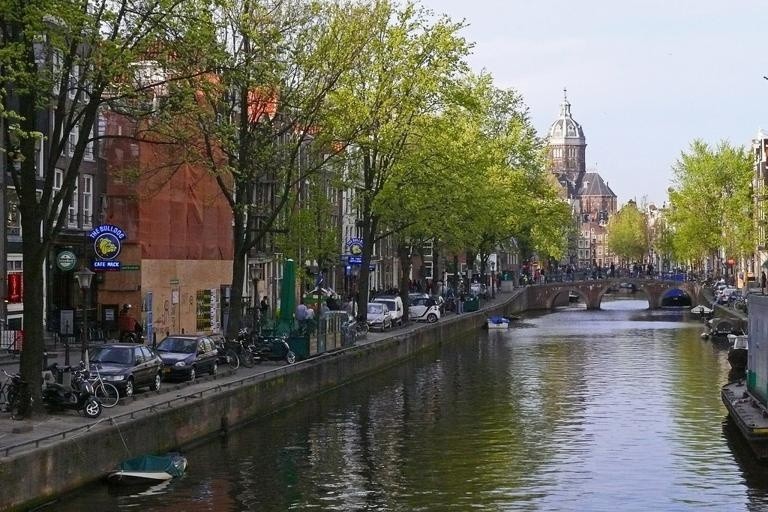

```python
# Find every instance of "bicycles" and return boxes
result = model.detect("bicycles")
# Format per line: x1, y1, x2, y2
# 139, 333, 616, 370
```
213, 327, 259, 370
52, 359, 120, 409
0, 368, 34, 422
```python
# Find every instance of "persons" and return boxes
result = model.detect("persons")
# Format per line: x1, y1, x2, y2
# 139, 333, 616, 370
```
261, 295, 270, 314
118, 303, 137, 340
294, 278, 432, 339
532, 259, 660, 287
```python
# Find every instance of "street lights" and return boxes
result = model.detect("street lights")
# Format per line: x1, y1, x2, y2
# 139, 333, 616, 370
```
251, 264, 264, 334
491, 261, 496, 299
316, 266, 328, 354
73, 267, 98, 370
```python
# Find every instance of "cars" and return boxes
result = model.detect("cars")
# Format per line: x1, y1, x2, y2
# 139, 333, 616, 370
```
71, 342, 165, 397
155, 334, 218, 381
713, 281, 737, 305
326, 280, 488, 338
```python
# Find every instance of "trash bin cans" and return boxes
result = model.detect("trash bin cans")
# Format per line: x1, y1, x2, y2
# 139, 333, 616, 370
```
288, 336, 309, 360
462, 294, 480, 312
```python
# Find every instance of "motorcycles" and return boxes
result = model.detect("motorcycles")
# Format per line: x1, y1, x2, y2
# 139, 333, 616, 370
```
121, 319, 147, 344
253, 334, 296, 364
42, 359, 103, 418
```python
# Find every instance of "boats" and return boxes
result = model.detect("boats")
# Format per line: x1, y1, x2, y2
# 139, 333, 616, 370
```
569, 294, 580, 303
486, 317, 510, 329
691, 305, 714, 314
734, 337, 748, 350
